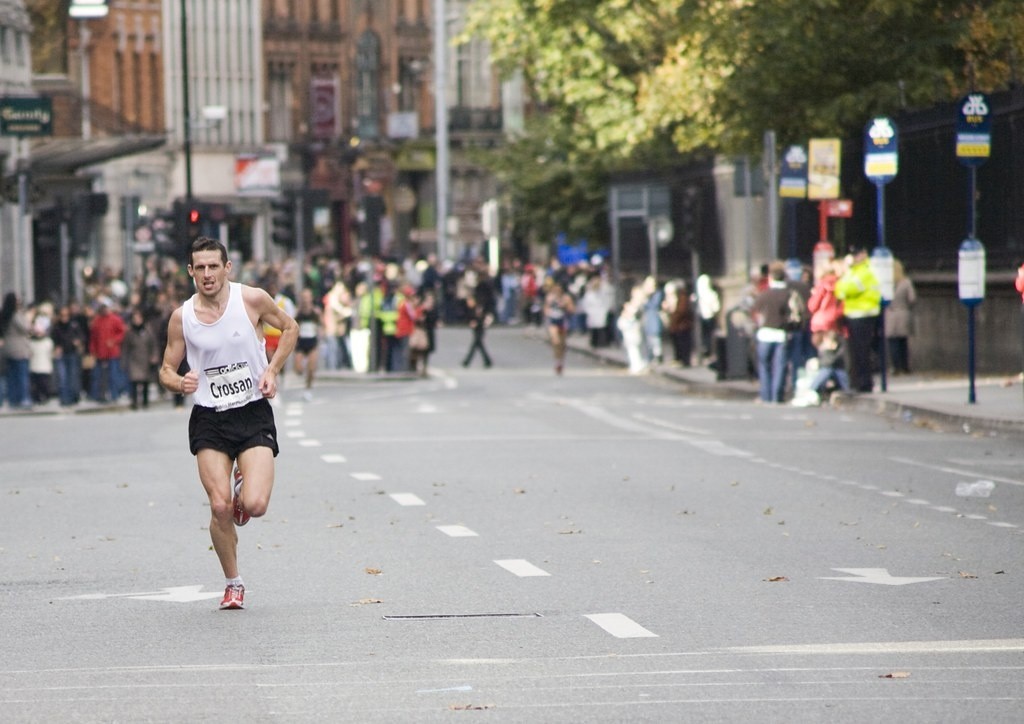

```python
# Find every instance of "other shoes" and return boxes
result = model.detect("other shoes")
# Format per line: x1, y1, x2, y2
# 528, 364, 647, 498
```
892, 365, 913, 377
790, 390, 820, 408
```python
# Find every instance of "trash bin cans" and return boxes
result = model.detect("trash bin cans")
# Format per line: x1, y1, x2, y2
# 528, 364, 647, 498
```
725, 305, 749, 378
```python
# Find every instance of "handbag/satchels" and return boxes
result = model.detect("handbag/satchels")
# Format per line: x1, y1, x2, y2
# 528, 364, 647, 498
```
410, 327, 429, 350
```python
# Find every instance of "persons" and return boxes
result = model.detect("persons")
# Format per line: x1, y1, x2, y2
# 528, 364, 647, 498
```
159, 237, 300, 610
0, 246, 915, 407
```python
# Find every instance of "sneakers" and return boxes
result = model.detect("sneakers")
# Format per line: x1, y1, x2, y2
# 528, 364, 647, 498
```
219, 582, 246, 609
228, 466, 251, 526
